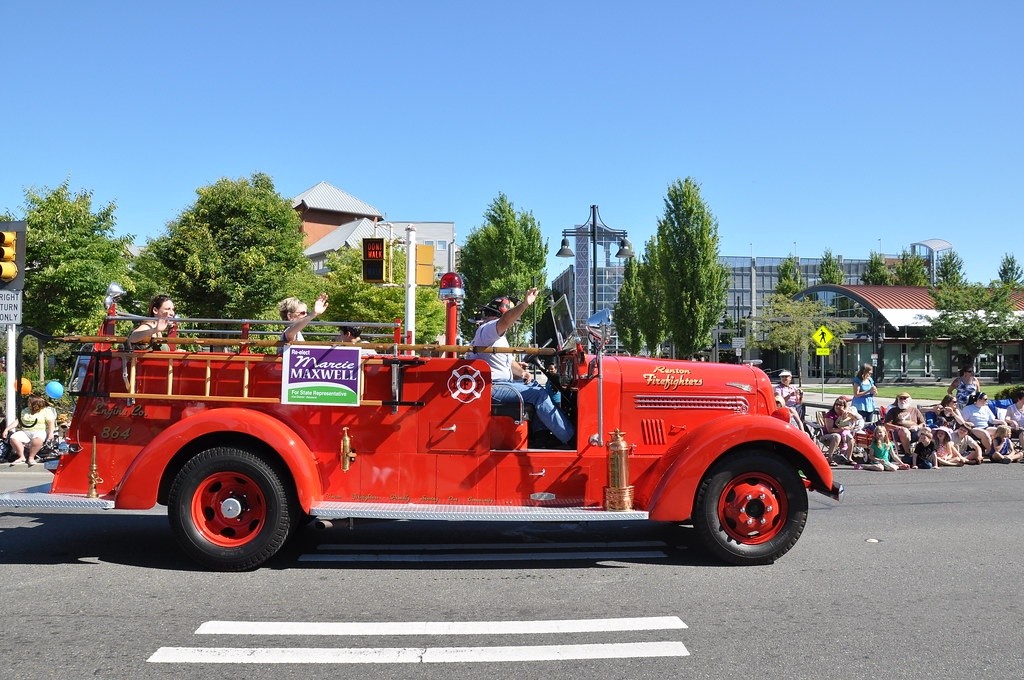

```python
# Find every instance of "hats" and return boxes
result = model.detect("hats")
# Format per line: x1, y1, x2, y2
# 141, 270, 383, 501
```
931, 426, 952, 441
840, 396, 852, 402
899, 392, 910, 397
480, 297, 516, 317
778, 372, 791, 376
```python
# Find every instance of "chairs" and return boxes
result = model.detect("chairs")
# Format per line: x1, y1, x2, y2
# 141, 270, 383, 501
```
926, 399, 1022, 454
23, 407, 61, 464
806, 405, 903, 464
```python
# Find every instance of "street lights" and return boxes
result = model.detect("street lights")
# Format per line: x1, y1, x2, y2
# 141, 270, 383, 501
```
557, 204, 636, 354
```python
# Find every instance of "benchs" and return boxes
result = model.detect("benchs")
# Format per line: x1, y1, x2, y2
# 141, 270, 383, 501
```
490, 381, 534, 451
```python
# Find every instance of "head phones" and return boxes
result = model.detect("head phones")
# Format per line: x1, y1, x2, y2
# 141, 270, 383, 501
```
476, 304, 502, 317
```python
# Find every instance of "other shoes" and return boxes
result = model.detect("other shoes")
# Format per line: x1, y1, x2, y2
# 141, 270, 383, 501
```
1001, 457, 1012, 464
854, 464, 862, 469
957, 460, 964, 467
905, 453, 913, 457
27, 459, 37, 466
11, 458, 27, 465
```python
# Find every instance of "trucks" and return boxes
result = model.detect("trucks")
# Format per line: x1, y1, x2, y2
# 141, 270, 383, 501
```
43, 269, 843, 577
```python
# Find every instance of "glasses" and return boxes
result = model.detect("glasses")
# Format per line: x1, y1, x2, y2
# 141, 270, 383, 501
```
900, 397, 908, 400
965, 370, 974, 373
952, 401, 958, 404
984, 398, 987, 401
835, 403, 844, 406
293, 311, 310, 316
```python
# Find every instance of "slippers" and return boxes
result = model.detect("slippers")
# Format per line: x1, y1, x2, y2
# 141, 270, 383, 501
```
846, 461, 857, 465
829, 462, 837, 466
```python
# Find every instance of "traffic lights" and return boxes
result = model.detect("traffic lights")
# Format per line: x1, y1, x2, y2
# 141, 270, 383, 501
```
361, 237, 389, 281
0, 226, 18, 286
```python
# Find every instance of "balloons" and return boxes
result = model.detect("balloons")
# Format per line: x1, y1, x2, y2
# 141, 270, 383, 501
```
45, 381, 64, 399
14, 378, 31, 394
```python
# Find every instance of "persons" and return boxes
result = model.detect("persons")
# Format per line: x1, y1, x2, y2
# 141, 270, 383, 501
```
773, 363, 1024, 471
122, 295, 186, 352
276, 292, 377, 355
466, 287, 577, 451
0, 391, 57, 466
